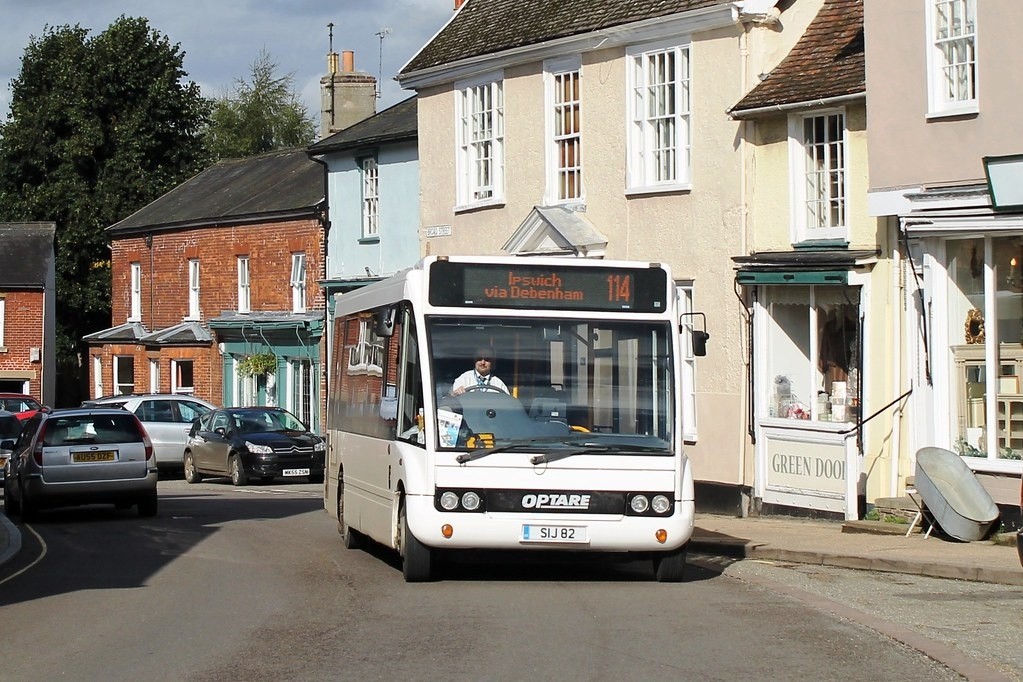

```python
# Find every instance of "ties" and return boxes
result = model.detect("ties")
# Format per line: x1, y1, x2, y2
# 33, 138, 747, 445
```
474, 370, 487, 392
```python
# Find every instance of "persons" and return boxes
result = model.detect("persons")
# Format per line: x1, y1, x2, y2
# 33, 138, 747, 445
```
449, 345, 510, 396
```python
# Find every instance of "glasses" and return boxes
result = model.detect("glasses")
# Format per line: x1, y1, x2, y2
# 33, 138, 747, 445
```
475, 356, 493, 362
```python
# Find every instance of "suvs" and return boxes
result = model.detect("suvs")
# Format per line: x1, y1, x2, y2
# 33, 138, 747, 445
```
0, 392, 43, 482
0, 402, 159, 524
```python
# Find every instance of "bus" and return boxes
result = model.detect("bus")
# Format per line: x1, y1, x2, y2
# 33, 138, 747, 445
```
319, 253, 709, 583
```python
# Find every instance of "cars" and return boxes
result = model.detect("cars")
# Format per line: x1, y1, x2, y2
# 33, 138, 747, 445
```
182, 406, 327, 486
63, 391, 244, 477
